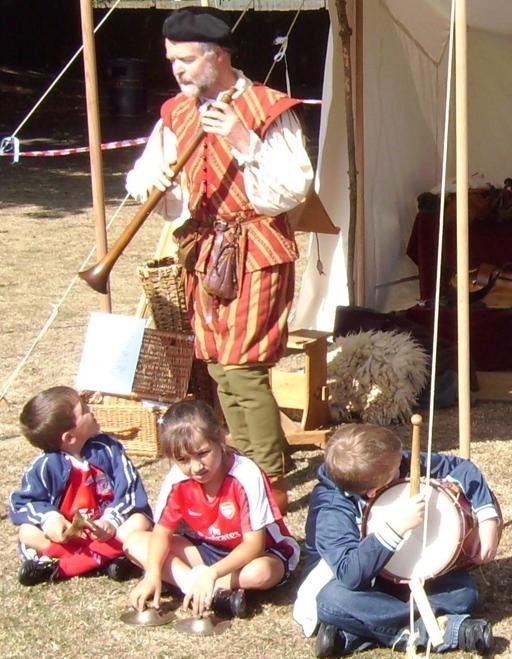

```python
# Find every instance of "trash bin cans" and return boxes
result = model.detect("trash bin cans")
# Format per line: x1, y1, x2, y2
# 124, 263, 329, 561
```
108, 57, 148, 120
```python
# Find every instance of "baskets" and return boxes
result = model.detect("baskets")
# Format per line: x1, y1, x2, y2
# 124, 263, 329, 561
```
89, 257, 216, 456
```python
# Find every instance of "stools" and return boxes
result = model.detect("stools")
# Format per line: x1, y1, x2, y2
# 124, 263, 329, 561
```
269, 329, 334, 432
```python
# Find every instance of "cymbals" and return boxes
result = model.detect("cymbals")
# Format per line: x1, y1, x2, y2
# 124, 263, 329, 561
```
176, 617, 230, 637
121, 608, 174, 628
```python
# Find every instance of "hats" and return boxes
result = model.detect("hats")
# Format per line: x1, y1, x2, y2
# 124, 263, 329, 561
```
163, 6, 238, 47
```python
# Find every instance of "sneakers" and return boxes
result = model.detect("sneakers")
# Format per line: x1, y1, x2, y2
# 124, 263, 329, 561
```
105, 556, 133, 579
458, 618, 492, 651
19, 560, 54, 586
213, 587, 246, 617
315, 621, 344, 658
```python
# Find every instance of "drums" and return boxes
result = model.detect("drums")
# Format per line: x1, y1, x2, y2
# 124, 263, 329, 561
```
362, 475, 503, 586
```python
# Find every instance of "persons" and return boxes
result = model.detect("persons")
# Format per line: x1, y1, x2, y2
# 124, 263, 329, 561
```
121, 398, 301, 618
9, 385, 153, 587
123, 5, 317, 480
293, 422, 504, 658
